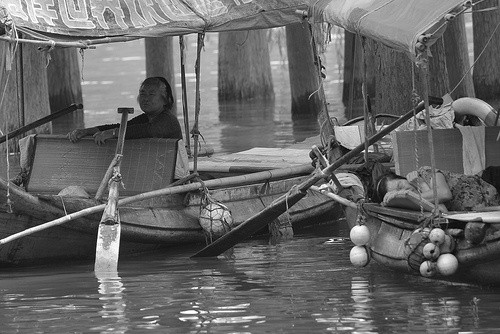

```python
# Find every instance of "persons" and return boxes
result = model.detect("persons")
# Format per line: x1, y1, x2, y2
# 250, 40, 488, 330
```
66, 77, 190, 185
377, 166, 500, 213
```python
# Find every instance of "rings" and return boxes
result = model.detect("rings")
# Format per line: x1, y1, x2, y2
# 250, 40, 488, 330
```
73, 131, 74, 133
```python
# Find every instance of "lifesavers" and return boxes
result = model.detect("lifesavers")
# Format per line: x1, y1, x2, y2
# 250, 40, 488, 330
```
452, 97, 500, 127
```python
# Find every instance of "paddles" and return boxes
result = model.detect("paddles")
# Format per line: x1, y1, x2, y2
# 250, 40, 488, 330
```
94, 107, 134, 272
189, 94, 443, 258
0, 103, 84, 144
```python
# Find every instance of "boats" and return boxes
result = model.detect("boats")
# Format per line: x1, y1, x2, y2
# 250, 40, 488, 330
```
1, 0, 344, 266
308, 1, 500, 289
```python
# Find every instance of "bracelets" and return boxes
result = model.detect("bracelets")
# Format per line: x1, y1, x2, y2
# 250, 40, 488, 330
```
112, 129, 116, 139
